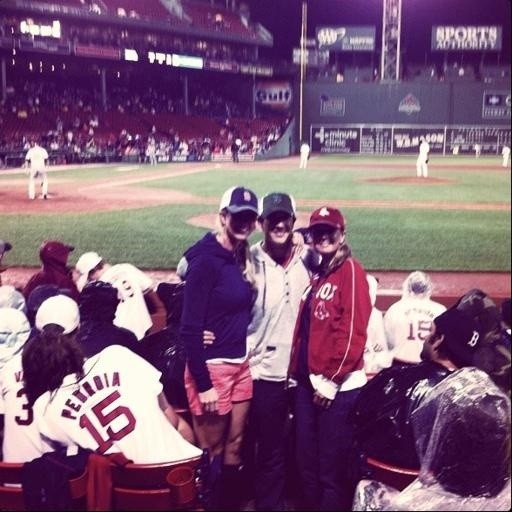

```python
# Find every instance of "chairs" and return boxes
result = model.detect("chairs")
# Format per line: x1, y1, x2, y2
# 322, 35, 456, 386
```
0, 106, 284, 152
88, 445, 208, 512
364, 454, 421, 492
0, 453, 97, 510
15, 1, 260, 68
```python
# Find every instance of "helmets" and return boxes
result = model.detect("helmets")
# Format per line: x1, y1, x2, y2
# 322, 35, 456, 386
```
79, 281, 121, 320
40, 243, 74, 266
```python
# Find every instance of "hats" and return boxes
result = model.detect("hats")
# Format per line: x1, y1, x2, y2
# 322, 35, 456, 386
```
311, 206, 345, 233
219, 186, 259, 215
36, 295, 80, 334
74, 251, 102, 292
262, 193, 297, 221
434, 308, 483, 359
28, 284, 70, 308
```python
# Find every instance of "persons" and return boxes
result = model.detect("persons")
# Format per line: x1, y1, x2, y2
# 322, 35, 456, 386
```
25, 141, 50, 199
0, 242, 511, 511
474, 143, 481, 158
501, 144, 510, 168
2, 27, 289, 164
174, 186, 262, 512
299, 140, 310, 169
284, 205, 373, 511
204, 192, 379, 511
416, 135, 430, 178
